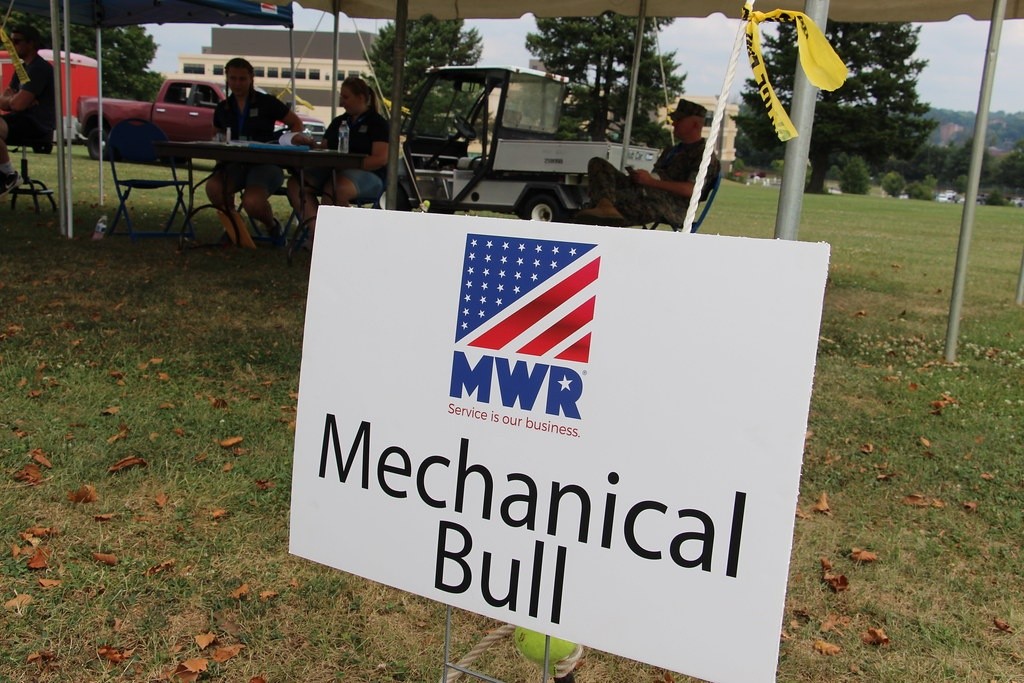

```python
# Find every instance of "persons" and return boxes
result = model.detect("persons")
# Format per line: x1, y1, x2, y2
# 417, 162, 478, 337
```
0, 24, 55, 196
205, 57, 304, 237
287, 77, 394, 246
577, 99, 720, 229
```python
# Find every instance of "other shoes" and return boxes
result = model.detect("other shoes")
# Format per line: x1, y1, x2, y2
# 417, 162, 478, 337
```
575, 198, 625, 223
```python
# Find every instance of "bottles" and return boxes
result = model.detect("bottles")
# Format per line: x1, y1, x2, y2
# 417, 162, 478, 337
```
411, 200, 432, 212
337, 121, 350, 154
92, 214, 108, 241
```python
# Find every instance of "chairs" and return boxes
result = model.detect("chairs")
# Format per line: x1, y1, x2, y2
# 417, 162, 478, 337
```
642, 174, 722, 232
104, 117, 195, 245
221, 175, 386, 250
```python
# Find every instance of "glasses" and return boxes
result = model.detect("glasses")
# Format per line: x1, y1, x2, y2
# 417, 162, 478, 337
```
9, 37, 24, 47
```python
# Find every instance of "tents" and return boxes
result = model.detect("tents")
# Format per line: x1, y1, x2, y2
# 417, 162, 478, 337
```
0, 0, 295, 236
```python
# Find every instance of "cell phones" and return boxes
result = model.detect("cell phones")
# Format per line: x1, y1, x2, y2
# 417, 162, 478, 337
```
625, 166, 634, 175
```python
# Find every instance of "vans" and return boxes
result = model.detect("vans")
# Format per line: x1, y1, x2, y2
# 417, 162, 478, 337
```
0, 47, 101, 153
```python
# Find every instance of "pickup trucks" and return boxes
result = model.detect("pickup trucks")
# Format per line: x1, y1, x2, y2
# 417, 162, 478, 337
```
76, 78, 327, 164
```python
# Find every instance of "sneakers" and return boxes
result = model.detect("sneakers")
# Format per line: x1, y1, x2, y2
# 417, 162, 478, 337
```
265, 217, 288, 249
0, 170, 24, 197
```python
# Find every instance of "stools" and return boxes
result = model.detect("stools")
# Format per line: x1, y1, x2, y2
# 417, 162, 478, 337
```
3, 137, 56, 215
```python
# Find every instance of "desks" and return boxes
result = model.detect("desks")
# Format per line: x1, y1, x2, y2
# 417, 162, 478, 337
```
153, 137, 369, 267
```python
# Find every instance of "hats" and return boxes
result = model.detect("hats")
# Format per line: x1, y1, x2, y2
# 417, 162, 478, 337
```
11, 24, 47, 49
667, 99, 707, 121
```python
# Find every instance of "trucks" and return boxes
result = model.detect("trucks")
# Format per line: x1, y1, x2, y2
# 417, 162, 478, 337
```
396, 65, 665, 222
